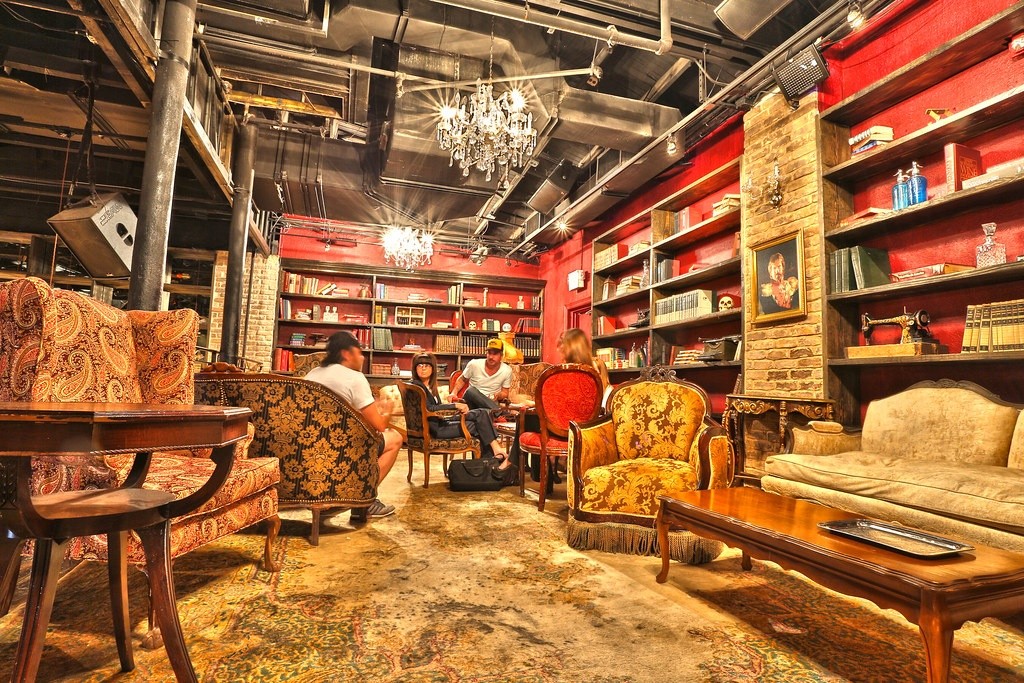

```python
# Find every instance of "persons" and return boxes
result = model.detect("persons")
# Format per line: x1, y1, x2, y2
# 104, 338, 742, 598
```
303, 332, 404, 519
492, 327, 609, 486
409, 352, 511, 460
447, 339, 514, 422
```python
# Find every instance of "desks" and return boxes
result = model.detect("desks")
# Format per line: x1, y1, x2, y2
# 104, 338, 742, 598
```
0, 395, 253, 681
722, 389, 836, 487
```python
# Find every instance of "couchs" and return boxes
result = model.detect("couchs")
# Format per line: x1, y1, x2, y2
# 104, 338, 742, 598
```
760, 378, 1023, 555
565, 364, 736, 565
0, 272, 288, 651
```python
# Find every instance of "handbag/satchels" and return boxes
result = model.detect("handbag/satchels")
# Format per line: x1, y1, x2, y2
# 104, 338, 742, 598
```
446, 459, 504, 492
489, 453, 525, 488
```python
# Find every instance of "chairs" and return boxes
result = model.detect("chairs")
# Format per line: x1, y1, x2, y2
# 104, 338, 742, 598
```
394, 379, 480, 488
192, 368, 384, 545
492, 360, 610, 512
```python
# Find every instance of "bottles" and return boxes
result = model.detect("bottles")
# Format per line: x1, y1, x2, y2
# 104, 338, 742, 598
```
517, 296, 524, 309
391, 358, 400, 375
629, 346, 638, 368
640, 259, 649, 288
323, 306, 339, 322
977, 222, 1006, 269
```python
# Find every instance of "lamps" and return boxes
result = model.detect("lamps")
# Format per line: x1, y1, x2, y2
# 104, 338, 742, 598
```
377, 16, 606, 266
666, 135, 680, 157
845, 1, 869, 32
770, 44, 830, 101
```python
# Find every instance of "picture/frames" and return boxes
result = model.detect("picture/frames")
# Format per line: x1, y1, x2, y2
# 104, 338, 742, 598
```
746, 227, 808, 325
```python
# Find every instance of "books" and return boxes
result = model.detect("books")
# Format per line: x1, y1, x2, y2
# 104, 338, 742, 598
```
828, 125, 1024, 353
273, 268, 540, 377
595, 192, 741, 370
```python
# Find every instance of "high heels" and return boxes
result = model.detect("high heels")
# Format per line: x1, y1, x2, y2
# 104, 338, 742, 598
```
491, 458, 519, 479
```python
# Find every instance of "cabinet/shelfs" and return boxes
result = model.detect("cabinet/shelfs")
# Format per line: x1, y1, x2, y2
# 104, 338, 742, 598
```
272, 258, 547, 387
815, 0, 1024, 432
590, 154, 743, 373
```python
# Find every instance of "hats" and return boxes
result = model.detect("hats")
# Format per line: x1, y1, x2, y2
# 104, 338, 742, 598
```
485, 339, 503, 351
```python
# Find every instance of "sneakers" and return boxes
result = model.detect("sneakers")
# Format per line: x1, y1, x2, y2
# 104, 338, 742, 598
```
350, 499, 396, 518
319, 506, 350, 516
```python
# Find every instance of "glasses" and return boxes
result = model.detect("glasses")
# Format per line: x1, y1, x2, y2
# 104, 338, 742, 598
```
417, 364, 432, 369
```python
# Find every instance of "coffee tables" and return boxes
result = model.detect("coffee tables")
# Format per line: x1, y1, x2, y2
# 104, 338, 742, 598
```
652, 486, 1023, 683
